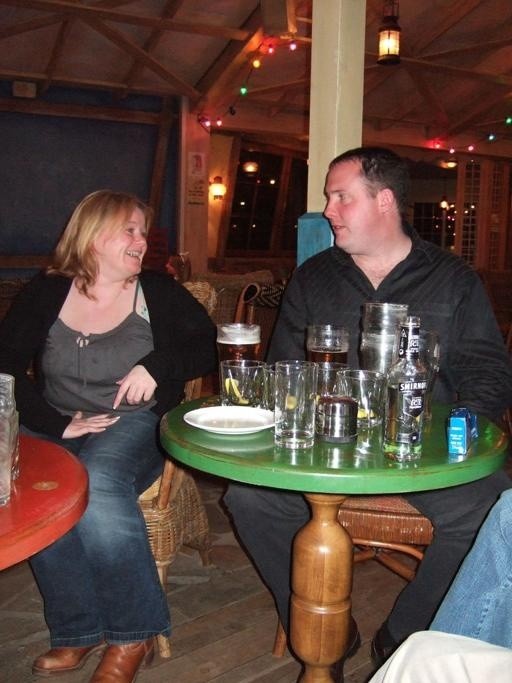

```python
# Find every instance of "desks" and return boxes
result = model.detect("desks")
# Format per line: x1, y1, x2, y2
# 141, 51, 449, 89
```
159, 394, 508, 682
0, 433, 91, 570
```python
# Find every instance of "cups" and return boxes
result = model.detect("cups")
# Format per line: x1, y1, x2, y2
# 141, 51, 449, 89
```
216, 302, 440, 449
0, 372, 22, 505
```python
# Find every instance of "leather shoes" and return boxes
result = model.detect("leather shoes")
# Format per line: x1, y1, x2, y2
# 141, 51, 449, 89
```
369, 623, 399, 669
297, 614, 361, 683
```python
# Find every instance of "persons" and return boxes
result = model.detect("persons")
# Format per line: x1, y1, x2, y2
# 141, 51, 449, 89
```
0, 190, 219, 683
367, 631, 512, 683
427, 489, 512, 648
221, 146, 512, 683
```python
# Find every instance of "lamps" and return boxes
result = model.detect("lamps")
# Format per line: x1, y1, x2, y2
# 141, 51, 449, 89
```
242, 153, 258, 178
207, 176, 229, 202
375, 0, 405, 67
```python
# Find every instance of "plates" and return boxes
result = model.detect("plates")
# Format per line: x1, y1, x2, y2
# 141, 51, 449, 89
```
183, 405, 278, 436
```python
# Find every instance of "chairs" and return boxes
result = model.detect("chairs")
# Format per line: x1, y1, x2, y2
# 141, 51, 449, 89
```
193, 270, 285, 399
272, 495, 436, 662
479, 263, 511, 351
0, 277, 20, 318
130, 281, 214, 599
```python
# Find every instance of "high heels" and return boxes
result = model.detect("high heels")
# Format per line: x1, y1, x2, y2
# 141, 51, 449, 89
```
31, 638, 106, 678
89, 636, 155, 682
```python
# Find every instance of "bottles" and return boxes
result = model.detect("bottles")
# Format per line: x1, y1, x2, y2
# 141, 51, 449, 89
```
385, 315, 428, 462
